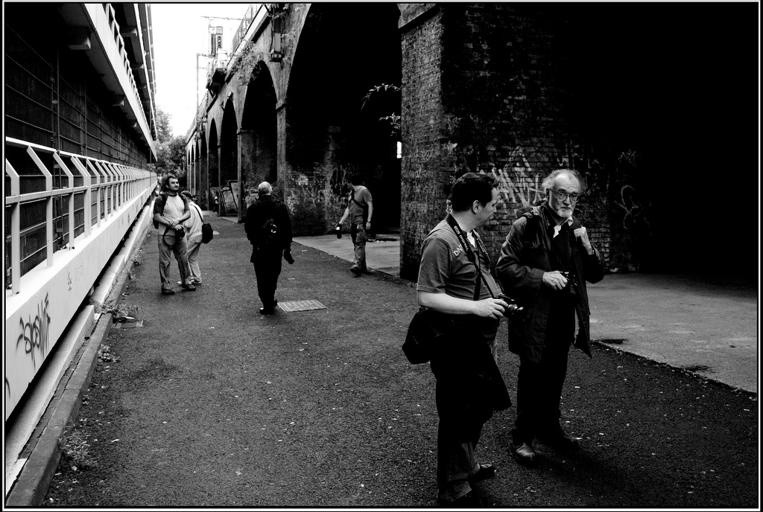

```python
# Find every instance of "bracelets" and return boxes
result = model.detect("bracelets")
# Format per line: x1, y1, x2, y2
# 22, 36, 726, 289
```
367, 221, 371, 223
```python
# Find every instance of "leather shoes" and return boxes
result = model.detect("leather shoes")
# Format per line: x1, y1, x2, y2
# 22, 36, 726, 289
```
438, 462, 496, 507
510, 428, 582, 465
259, 298, 277, 314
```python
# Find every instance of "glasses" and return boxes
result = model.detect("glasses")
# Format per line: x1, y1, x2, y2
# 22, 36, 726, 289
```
551, 189, 580, 202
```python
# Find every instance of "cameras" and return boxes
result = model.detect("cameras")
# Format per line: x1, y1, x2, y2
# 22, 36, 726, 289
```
560, 271, 580, 298
496, 294, 524, 321
175, 228, 184, 238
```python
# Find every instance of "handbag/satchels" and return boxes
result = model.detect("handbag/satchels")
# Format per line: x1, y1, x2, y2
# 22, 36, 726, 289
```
202, 223, 213, 244
402, 307, 440, 365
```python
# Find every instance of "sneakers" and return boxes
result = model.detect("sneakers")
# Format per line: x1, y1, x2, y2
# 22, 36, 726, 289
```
162, 285, 176, 294
350, 261, 367, 276
177, 276, 201, 290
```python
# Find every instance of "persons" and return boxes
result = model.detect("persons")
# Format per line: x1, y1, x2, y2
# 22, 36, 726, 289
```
335, 174, 373, 277
153, 175, 196, 295
416, 171, 505, 509
176, 190, 204, 286
244, 181, 293, 315
496, 169, 607, 464
